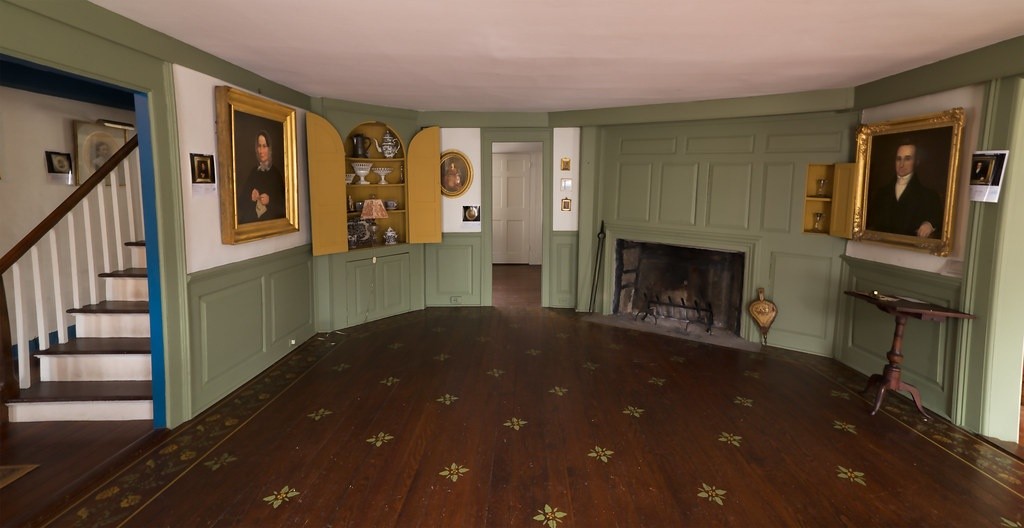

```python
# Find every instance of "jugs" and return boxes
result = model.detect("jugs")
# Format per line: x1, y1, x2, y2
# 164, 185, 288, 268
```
351, 133, 371, 158
383, 226, 399, 245
348, 234, 358, 249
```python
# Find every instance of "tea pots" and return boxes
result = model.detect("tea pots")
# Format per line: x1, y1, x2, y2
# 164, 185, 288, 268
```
373, 130, 400, 159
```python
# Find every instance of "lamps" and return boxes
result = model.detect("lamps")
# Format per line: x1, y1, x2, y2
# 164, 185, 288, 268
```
360, 193, 389, 246
97, 119, 134, 130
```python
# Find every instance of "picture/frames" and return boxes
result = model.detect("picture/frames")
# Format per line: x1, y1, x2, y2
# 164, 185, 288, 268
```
45, 150, 72, 174
215, 86, 300, 244
74, 120, 129, 186
854, 107, 965, 257
190, 153, 215, 184
970, 156, 997, 186
438, 150, 473, 199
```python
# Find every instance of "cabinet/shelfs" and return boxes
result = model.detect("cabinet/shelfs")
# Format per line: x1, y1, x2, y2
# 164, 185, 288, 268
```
307, 110, 481, 334
593, 109, 856, 357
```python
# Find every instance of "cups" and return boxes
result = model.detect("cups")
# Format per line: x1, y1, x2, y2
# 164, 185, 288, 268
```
387, 200, 396, 208
355, 201, 363, 211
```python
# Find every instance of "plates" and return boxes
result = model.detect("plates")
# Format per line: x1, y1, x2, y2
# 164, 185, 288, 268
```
386, 207, 398, 210
354, 217, 373, 243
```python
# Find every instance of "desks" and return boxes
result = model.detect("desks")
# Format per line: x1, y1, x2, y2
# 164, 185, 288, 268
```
844, 294, 977, 422
